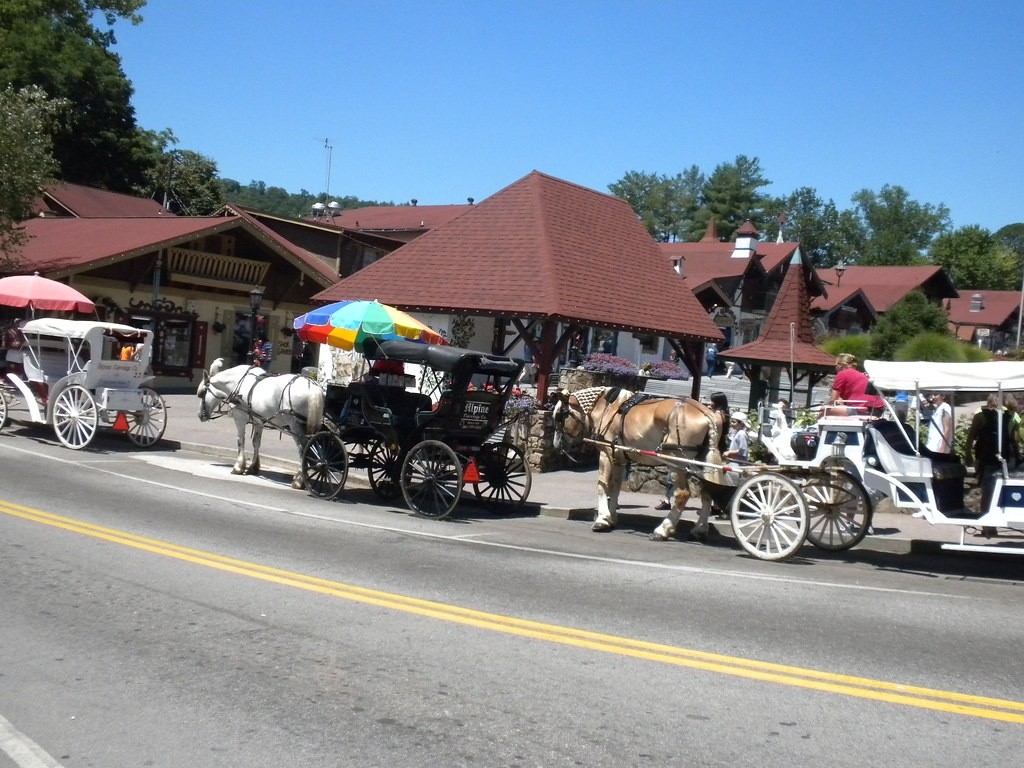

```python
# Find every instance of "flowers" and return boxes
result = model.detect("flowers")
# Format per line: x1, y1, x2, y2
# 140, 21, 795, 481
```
582, 352, 639, 375
469, 383, 556, 410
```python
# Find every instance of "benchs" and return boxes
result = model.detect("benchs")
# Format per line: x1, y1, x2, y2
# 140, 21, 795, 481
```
866, 415, 967, 477
6, 339, 74, 363
23, 340, 85, 382
349, 380, 434, 424
70, 359, 149, 386
817, 400, 878, 460
980, 436, 1024, 479
416, 392, 509, 424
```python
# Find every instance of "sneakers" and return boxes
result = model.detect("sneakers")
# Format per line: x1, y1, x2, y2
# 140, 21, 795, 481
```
654, 501, 672, 510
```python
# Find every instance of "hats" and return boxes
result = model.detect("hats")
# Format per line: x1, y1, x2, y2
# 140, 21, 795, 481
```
731, 412, 751, 430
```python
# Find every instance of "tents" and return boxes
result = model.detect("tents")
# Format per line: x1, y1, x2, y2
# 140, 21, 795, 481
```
864, 359, 1024, 410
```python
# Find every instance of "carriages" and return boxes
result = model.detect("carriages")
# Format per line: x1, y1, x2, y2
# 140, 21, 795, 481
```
0, 317, 167, 450
196, 337, 532, 522
550, 358, 1024, 564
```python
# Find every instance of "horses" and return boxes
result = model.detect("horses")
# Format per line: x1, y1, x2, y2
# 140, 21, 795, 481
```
196, 364, 325, 492
551, 386, 723, 542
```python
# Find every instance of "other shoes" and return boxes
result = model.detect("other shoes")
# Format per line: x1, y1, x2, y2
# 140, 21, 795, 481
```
715, 514, 729, 520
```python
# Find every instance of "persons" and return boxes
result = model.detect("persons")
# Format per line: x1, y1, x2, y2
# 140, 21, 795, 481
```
706, 343, 719, 378
6, 318, 24, 378
925, 390, 953, 454
724, 346, 736, 379
655, 392, 751, 520
994, 344, 1010, 357
257, 338, 272, 370
372, 359, 405, 390
120, 342, 143, 361
817, 353, 885, 420
965, 393, 1024, 514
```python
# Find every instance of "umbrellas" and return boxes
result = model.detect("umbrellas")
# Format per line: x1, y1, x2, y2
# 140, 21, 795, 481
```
293, 299, 448, 382
0, 271, 95, 318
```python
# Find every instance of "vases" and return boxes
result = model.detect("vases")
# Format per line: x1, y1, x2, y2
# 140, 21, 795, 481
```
509, 410, 589, 473
559, 368, 650, 394
